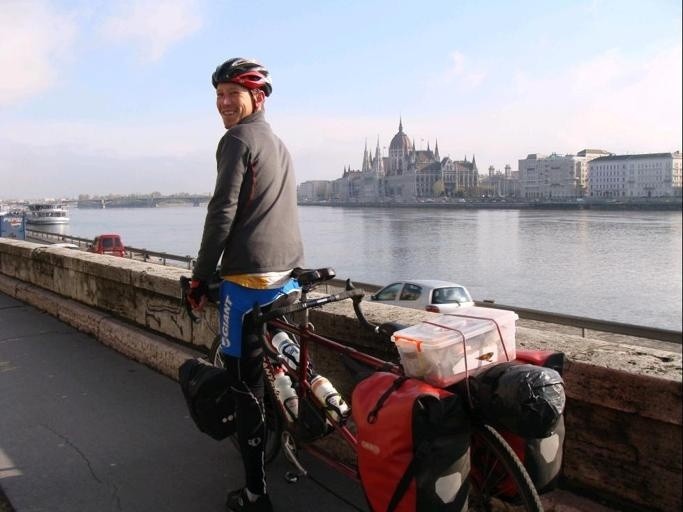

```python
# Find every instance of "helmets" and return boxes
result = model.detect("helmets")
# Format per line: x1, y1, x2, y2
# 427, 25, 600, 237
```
211, 56, 274, 98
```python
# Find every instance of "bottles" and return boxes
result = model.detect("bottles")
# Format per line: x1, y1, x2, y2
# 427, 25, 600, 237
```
269, 328, 347, 424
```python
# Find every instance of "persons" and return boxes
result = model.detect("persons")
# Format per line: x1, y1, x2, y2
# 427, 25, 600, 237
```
187, 57, 354, 509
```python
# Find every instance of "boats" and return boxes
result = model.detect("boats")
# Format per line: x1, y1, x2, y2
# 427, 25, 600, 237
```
25, 203, 70, 242
8, 218, 22, 228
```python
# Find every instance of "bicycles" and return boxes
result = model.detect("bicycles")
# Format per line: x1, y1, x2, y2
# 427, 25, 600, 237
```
180, 267, 543, 512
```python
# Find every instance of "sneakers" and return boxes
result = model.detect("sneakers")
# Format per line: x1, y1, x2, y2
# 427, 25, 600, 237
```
224, 487, 275, 511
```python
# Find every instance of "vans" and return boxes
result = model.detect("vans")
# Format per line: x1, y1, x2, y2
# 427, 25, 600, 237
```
86, 235, 125, 257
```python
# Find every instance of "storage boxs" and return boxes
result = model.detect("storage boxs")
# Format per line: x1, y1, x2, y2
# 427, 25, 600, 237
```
390, 305, 519, 389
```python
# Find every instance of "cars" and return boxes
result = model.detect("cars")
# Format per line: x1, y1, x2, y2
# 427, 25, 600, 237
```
52, 243, 79, 250
368, 279, 475, 314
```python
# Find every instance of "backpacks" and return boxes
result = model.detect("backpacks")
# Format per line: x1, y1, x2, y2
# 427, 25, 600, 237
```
177, 357, 251, 443
351, 371, 473, 511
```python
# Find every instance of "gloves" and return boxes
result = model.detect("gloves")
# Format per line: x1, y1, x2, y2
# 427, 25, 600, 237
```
188, 280, 208, 300
